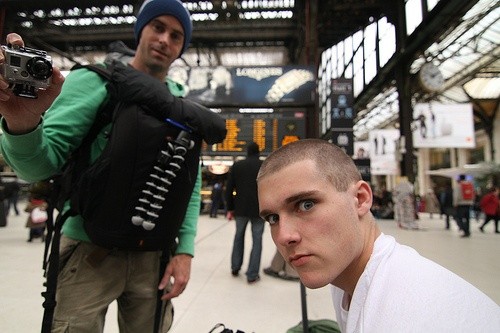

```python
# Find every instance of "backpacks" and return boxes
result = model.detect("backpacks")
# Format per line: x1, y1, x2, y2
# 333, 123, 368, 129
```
68, 60, 203, 255
460, 181, 474, 200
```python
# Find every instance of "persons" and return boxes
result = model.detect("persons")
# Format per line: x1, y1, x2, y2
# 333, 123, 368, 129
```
0, 177, 19, 227
0, 1, 201, 333
209, 183, 224, 218
26, 182, 46, 243
257, 139, 500, 333
426, 178, 443, 220
440, 173, 474, 238
224, 140, 265, 284
371, 172, 419, 230
473, 186, 500, 234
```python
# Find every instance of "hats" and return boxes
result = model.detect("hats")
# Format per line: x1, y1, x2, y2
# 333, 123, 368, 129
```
133, 0, 193, 59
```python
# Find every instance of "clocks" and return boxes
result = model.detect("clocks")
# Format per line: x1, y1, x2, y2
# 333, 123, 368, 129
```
418, 63, 444, 92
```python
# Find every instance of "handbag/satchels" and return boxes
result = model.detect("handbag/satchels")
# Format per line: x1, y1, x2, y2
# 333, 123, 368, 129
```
32, 207, 48, 223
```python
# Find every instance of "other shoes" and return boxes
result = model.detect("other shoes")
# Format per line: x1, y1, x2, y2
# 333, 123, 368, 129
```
231, 270, 238, 276
248, 277, 257, 283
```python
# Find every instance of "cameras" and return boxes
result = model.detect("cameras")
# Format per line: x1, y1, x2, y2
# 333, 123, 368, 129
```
1, 44, 53, 100
